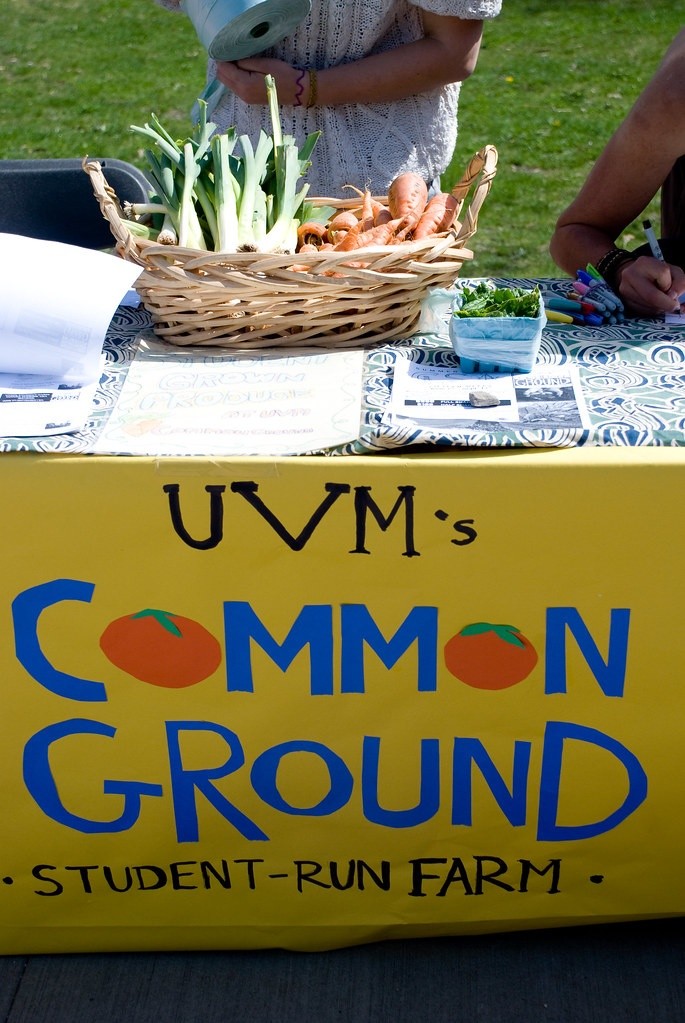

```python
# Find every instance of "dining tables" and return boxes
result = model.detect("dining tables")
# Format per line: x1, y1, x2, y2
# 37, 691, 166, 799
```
2, 230, 684, 1023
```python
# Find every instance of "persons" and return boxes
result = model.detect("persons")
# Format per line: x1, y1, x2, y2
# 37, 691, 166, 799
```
154, 0, 506, 207
546, 25, 685, 324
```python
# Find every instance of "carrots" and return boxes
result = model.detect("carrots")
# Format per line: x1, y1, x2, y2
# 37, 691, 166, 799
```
291, 169, 460, 279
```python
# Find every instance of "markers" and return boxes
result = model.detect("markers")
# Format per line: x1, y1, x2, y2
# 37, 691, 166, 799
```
538, 263, 627, 325
640, 219, 681, 317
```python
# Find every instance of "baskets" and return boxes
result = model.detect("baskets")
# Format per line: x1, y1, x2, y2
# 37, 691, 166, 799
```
82, 137, 500, 349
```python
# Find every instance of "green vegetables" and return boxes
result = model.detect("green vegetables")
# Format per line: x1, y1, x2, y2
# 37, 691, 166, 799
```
455, 282, 540, 318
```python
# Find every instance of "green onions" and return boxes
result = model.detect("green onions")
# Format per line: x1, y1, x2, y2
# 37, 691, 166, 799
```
121, 73, 322, 255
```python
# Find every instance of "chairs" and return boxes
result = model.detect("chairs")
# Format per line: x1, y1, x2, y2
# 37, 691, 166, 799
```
1, 158, 154, 253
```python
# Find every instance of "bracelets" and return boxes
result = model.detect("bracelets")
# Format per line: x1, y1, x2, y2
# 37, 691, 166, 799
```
306, 68, 320, 109
293, 66, 306, 107
594, 248, 635, 293
